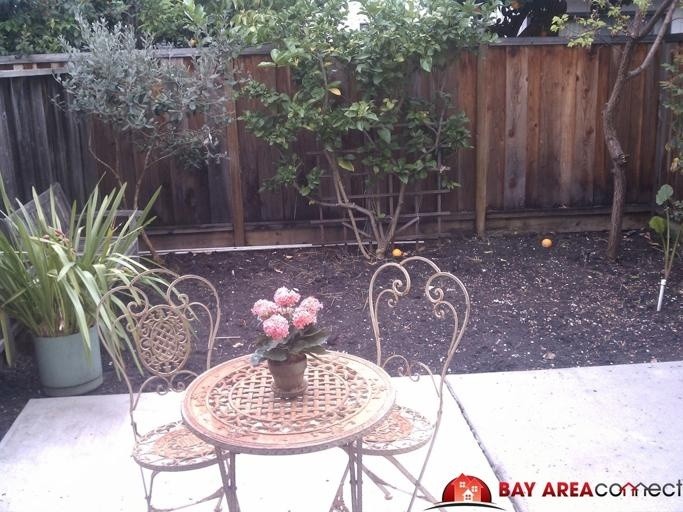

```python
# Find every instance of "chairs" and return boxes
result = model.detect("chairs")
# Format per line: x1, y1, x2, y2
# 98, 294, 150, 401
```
93, 265, 240, 512
327, 254, 473, 512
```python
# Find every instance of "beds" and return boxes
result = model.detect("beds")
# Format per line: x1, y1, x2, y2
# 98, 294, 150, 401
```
181, 347, 398, 512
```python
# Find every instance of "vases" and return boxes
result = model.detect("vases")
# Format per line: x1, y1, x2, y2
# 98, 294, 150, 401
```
263, 355, 312, 399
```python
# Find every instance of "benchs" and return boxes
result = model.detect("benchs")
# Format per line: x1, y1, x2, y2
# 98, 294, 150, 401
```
1, 181, 148, 296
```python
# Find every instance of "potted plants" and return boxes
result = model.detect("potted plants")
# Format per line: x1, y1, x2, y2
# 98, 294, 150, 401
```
0, 172, 176, 398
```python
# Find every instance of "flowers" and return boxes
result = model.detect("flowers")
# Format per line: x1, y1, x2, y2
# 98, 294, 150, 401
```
250, 281, 330, 370
